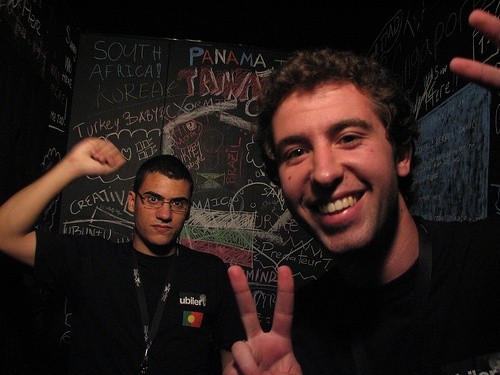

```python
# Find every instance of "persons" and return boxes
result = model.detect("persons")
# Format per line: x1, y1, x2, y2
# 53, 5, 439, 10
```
222, 3, 500, 375
0, 137, 249, 375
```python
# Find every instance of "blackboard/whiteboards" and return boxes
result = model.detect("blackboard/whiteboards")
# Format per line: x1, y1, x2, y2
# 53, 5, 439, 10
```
0, 0, 500, 338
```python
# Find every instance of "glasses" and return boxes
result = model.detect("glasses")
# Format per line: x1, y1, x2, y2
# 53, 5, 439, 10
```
136, 190, 190, 212
